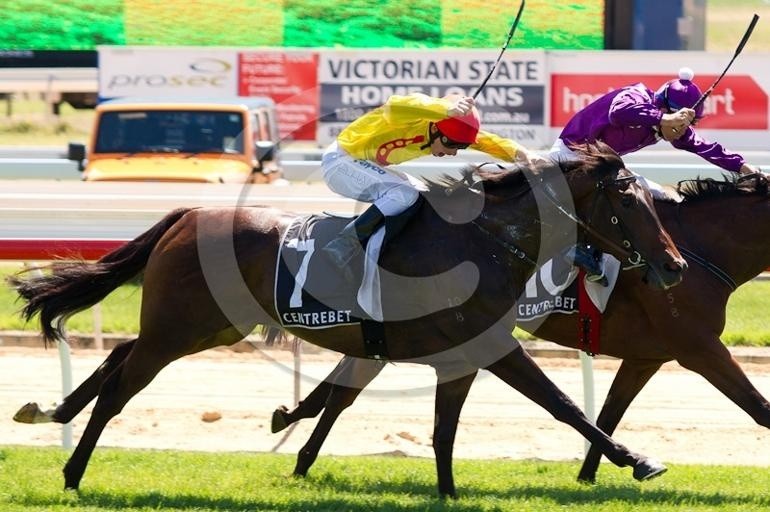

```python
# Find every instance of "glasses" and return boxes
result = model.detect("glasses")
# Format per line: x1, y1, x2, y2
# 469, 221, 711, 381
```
440, 134, 469, 149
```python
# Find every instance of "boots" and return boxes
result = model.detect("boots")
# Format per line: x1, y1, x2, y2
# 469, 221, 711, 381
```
326, 204, 385, 282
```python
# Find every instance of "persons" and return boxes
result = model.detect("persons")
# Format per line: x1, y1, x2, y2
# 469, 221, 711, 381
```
545, 68, 766, 286
319, 91, 551, 270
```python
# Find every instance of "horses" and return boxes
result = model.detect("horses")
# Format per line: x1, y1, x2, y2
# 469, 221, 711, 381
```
5, 139, 690, 507
257, 169, 770, 486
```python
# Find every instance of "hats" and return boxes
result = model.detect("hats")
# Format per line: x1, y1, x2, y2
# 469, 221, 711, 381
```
654, 68, 704, 119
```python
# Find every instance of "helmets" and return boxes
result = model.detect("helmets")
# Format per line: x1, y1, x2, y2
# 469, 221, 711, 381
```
436, 106, 480, 144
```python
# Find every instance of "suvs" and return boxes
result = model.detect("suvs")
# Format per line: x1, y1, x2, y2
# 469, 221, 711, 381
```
68, 99, 286, 188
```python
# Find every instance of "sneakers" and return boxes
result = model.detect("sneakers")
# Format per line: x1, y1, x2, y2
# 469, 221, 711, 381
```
564, 243, 608, 288
